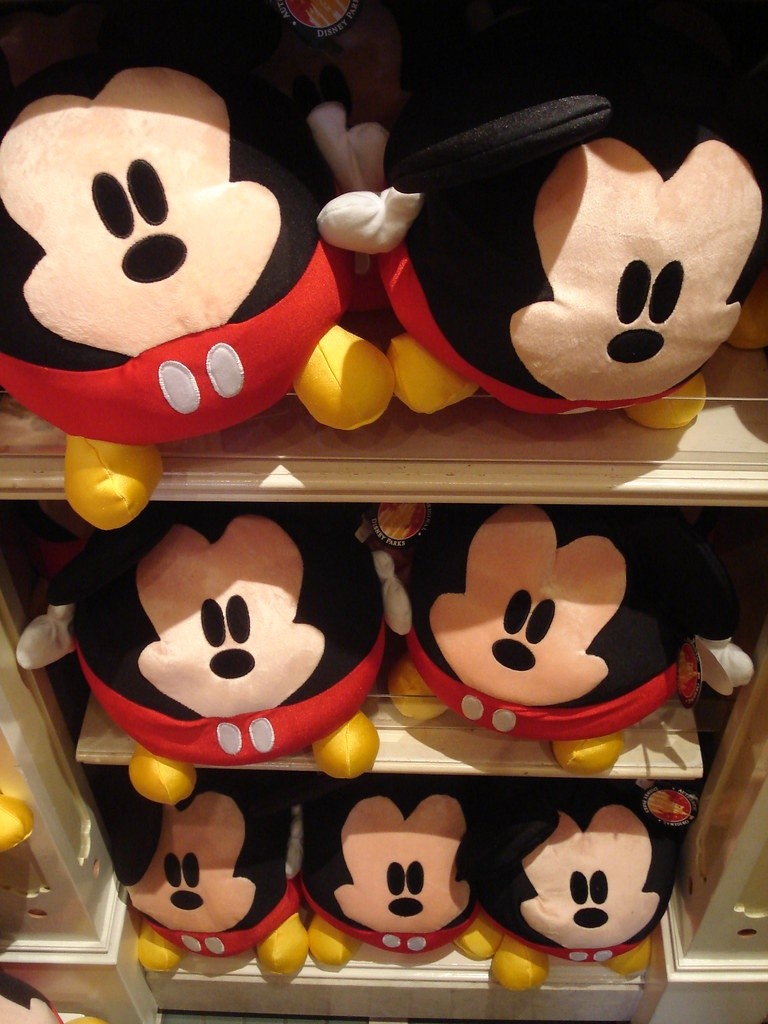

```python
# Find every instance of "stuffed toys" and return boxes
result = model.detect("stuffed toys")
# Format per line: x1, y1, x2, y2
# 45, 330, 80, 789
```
455, 777, 680, 991
297, 774, 504, 971
314, 76, 766, 444
15, 504, 393, 790
2, 0, 392, 530
387, 504, 754, 776
100, 765, 310, 979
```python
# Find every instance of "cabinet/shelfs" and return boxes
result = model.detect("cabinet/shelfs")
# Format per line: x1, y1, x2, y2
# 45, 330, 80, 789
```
0, 341, 768, 1023
